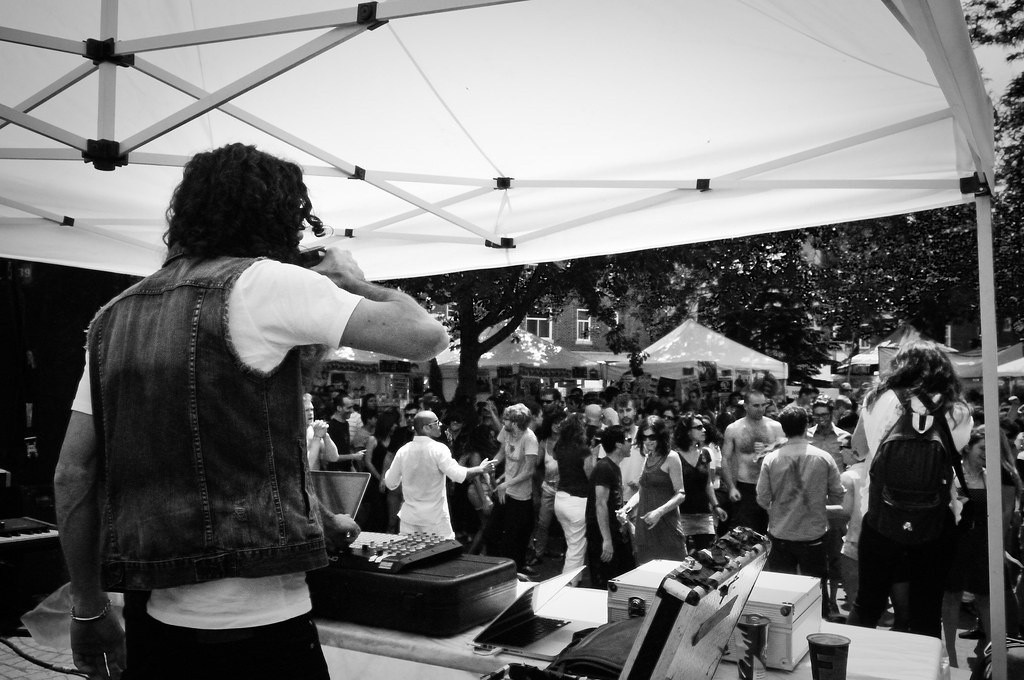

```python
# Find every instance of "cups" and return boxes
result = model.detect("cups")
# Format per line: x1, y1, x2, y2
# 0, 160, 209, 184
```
806, 632, 852, 680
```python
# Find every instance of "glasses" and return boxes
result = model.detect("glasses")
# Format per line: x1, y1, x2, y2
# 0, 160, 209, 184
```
748, 403, 766, 407
689, 425, 704, 430
622, 438, 632, 442
812, 413, 830, 419
428, 420, 439, 426
641, 434, 658, 441
842, 389, 853, 392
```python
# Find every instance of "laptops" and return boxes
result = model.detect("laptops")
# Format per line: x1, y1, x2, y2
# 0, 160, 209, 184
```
310, 471, 371, 520
469, 564, 604, 663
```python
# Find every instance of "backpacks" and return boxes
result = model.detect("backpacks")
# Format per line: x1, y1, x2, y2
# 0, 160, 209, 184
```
869, 384, 953, 514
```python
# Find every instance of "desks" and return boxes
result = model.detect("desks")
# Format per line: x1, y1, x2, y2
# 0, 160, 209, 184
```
109, 572, 950, 680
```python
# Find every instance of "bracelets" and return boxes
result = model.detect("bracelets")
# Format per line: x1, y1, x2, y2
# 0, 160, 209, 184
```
712, 504, 721, 507
70, 599, 113, 622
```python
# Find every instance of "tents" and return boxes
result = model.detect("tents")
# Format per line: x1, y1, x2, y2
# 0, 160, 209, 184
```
845, 324, 1024, 386
0, 1, 1008, 680
602, 316, 789, 397
428, 317, 594, 386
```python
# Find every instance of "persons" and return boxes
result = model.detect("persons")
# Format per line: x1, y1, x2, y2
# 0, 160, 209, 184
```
53, 143, 450, 679
296, 342, 1024, 641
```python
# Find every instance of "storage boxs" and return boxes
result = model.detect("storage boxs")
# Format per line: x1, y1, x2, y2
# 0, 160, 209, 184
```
607, 558, 823, 672
531, 527, 771, 680
307, 536, 520, 638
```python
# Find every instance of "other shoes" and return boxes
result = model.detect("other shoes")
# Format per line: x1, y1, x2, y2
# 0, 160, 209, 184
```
519, 566, 539, 577
876, 611, 895, 627
958, 625, 986, 639
